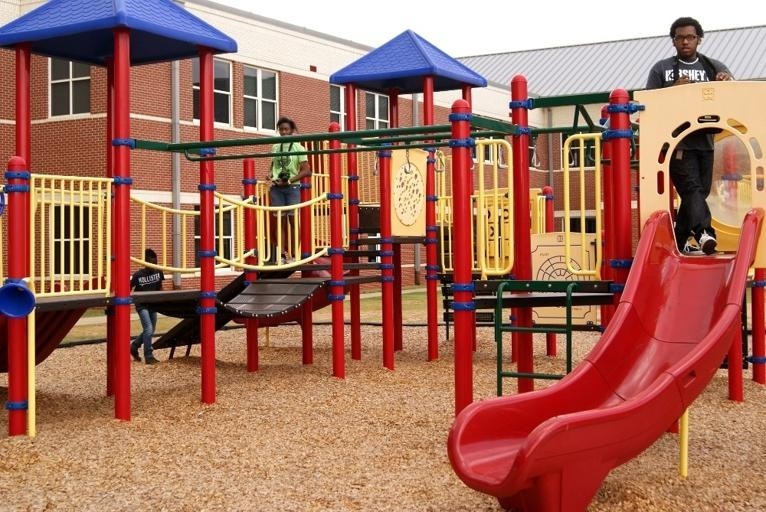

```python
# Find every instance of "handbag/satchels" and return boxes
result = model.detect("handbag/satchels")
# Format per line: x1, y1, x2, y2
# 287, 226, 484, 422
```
674, 53, 724, 136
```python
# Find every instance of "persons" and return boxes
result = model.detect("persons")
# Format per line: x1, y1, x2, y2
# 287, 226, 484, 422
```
130, 250, 168, 362
269, 115, 311, 262
645, 17, 736, 255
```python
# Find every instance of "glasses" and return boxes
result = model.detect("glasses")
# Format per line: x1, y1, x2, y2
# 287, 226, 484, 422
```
673, 34, 698, 43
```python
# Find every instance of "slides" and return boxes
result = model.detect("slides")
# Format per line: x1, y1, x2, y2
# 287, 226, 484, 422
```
446, 208, 763, 510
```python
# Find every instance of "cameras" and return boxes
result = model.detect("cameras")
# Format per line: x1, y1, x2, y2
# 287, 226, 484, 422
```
279, 172, 290, 182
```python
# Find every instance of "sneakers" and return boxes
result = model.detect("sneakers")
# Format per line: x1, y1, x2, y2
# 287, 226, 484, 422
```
681, 240, 702, 255
131, 343, 142, 362
699, 233, 718, 256
144, 356, 160, 364
